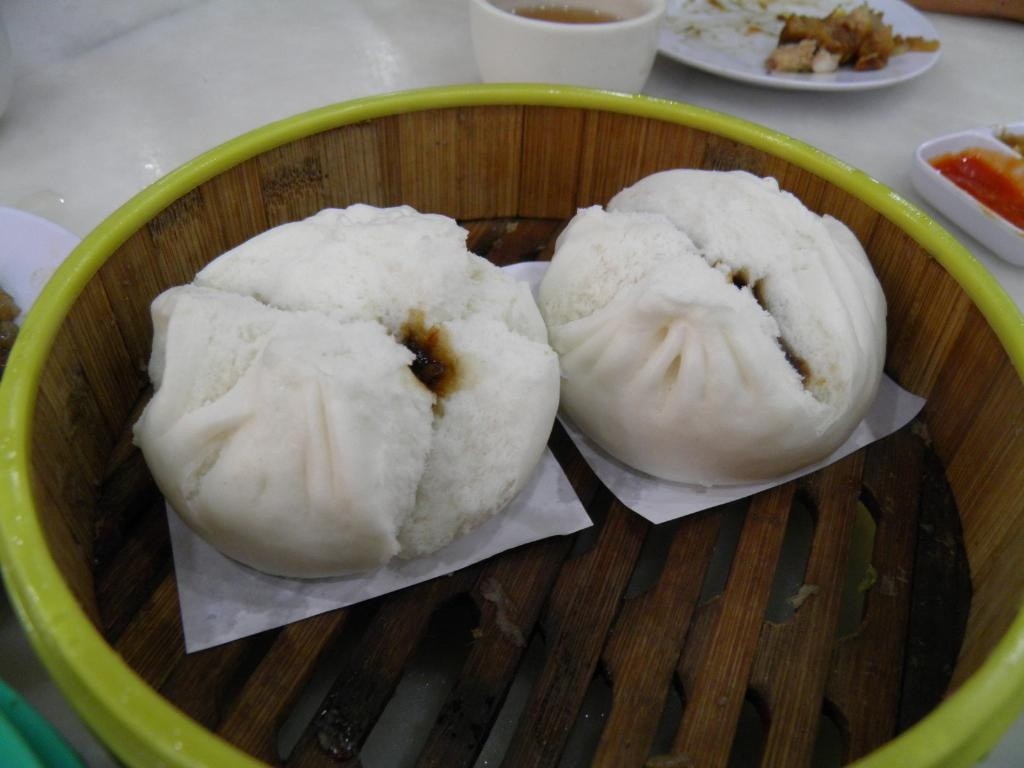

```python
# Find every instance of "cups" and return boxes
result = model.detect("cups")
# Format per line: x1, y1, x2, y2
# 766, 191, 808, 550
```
473, 0, 657, 93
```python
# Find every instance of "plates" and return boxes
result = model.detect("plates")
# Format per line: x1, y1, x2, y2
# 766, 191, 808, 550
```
912, 122, 1024, 267
0, 207, 82, 364
657, 0, 943, 91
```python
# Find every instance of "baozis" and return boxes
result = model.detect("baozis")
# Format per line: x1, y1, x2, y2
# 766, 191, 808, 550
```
132, 202, 559, 577
539, 168, 888, 486
